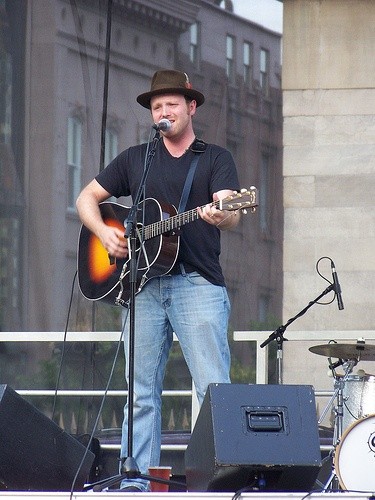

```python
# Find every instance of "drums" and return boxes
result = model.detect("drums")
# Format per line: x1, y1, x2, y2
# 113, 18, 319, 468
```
333, 412, 375, 494
329, 369, 375, 474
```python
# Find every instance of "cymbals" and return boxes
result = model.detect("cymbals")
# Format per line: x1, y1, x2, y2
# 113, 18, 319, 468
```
309, 343, 375, 362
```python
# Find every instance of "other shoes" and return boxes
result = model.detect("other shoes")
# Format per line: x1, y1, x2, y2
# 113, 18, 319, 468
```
120, 486, 142, 492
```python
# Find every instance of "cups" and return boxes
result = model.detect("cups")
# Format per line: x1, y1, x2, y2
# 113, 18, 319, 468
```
147, 466, 172, 493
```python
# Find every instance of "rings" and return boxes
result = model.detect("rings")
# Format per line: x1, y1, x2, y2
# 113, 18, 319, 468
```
209, 215, 215, 219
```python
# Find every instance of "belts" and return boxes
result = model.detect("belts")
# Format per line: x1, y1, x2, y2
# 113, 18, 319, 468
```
168, 263, 195, 276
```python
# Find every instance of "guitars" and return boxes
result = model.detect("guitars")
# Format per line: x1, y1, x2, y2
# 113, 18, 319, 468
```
77, 183, 262, 305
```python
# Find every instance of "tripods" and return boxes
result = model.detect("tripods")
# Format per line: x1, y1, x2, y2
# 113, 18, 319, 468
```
312, 356, 373, 493
84, 128, 188, 492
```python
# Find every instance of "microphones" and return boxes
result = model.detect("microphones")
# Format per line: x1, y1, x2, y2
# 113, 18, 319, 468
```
331, 261, 343, 308
151, 118, 171, 131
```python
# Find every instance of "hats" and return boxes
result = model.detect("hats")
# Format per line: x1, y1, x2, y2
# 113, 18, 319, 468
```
137, 69, 205, 111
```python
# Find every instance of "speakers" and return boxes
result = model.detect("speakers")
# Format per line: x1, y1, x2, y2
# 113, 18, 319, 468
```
183, 383, 321, 493
0, 386, 95, 491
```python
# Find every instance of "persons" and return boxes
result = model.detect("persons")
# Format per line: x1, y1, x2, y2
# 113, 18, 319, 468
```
76, 70, 240, 492
